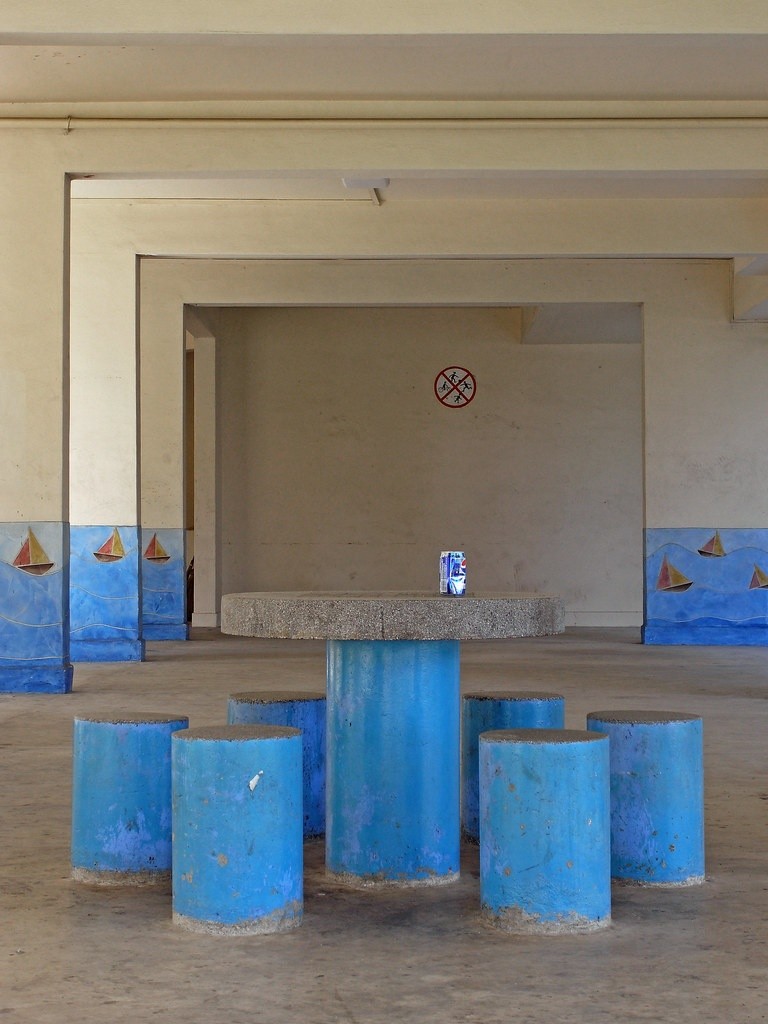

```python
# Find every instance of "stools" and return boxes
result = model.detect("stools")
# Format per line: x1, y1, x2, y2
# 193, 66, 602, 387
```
461, 692, 565, 841
171, 726, 304, 934
587, 710, 705, 886
71, 714, 188, 887
478, 730, 612, 935
227, 691, 327, 843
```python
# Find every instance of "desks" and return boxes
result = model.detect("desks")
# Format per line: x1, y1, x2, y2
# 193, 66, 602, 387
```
222, 591, 565, 887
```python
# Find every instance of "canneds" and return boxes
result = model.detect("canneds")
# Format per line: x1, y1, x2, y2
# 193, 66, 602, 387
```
439, 551, 467, 596
